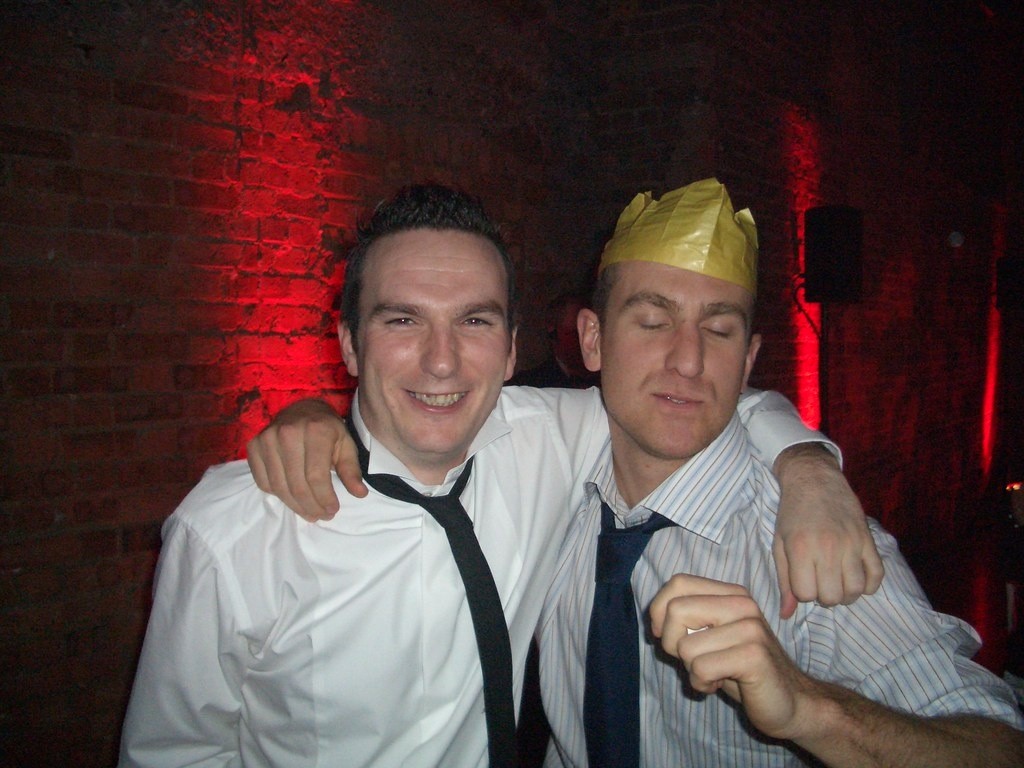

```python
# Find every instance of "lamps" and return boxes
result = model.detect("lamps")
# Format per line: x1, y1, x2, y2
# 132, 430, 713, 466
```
804, 203, 863, 303
995, 253, 1024, 315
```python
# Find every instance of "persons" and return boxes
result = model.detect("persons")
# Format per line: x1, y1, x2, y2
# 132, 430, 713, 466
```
119, 185, 887, 768
246, 177, 1024, 768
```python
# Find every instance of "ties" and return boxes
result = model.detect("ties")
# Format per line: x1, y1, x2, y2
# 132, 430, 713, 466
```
342, 403, 516, 768
583, 501, 679, 768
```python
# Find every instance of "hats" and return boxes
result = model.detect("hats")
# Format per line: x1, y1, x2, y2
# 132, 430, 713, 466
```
596, 177, 759, 299
